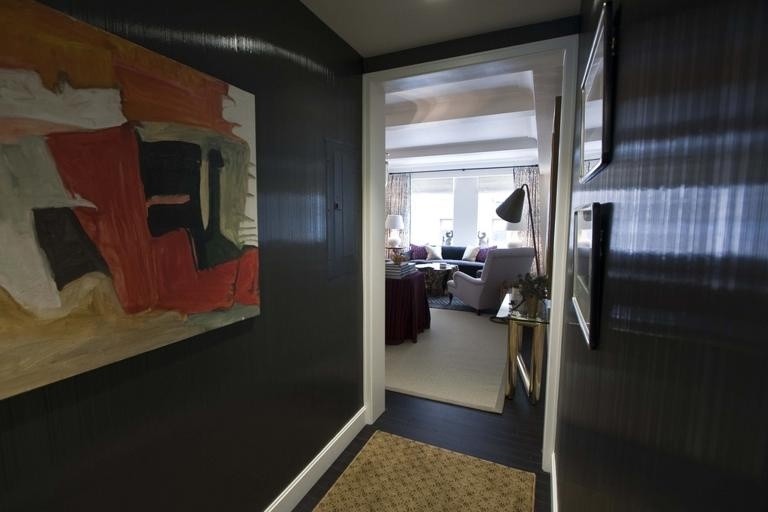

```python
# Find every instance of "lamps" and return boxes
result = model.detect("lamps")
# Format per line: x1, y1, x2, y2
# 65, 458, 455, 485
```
385, 214, 404, 247
496, 184, 541, 276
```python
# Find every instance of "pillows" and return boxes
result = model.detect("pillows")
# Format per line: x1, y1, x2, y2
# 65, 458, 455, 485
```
409, 244, 496, 261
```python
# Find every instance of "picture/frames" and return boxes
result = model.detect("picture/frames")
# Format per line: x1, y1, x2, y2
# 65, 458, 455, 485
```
571, 202, 600, 347
579, 6, 610, 184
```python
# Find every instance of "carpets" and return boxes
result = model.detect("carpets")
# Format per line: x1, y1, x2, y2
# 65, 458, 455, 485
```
312, 429, 536, 511
384, 308, 508, 414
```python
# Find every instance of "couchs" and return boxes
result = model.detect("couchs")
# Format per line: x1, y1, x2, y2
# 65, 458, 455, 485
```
400, 242, 497, 278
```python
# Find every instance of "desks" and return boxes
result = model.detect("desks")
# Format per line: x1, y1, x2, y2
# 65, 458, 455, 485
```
385, 271, 431, 345
490, 298, 550, 406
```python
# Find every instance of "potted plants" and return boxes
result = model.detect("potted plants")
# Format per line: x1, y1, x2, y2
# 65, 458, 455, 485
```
517, 273, 549, 318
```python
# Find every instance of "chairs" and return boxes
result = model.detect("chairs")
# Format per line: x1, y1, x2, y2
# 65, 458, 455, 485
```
447, 247, 537, 316
